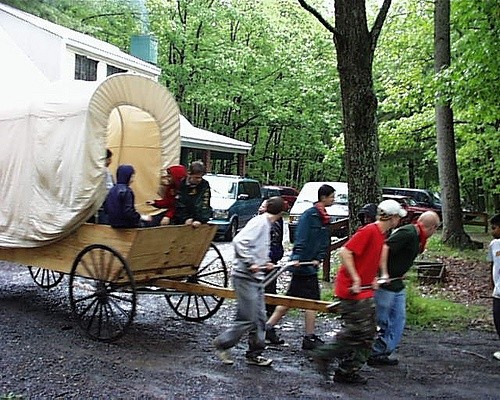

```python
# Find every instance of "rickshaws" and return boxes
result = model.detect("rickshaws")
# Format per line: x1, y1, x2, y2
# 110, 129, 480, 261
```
0, 74, 411, 342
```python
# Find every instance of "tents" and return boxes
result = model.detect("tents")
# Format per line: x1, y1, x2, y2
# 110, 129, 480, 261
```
1, 73, 181, 248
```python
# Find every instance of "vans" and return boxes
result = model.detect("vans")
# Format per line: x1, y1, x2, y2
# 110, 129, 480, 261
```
263, 185, 299, 209
202, 174, 263, 240
381, 187, 469, 224
289, 182, 351, 241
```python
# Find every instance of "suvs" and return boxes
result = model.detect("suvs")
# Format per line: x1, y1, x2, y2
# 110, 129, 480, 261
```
382, 196, 440, 223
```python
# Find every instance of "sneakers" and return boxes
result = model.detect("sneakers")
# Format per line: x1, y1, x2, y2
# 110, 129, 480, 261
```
309, 354, 331, 381
244, 355, 272, 365
368, 354, 399, 365
299, 334, 324, 349
211, 338, 234, 364
493, 351, 500, 359
333, 369, 368, 384
266, 327, 285, 343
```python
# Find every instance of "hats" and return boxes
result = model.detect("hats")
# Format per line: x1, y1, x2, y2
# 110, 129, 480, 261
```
376, 199, 407, 221
187, 160, 207, 175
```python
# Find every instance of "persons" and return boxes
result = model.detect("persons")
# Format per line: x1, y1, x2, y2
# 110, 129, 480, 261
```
489, 214, 500, 360
310, 199, 407, 383
212, 196, 288, 366
266, 185, 336, 349
101, 149, 211, 229
368, 210, 440, 365
